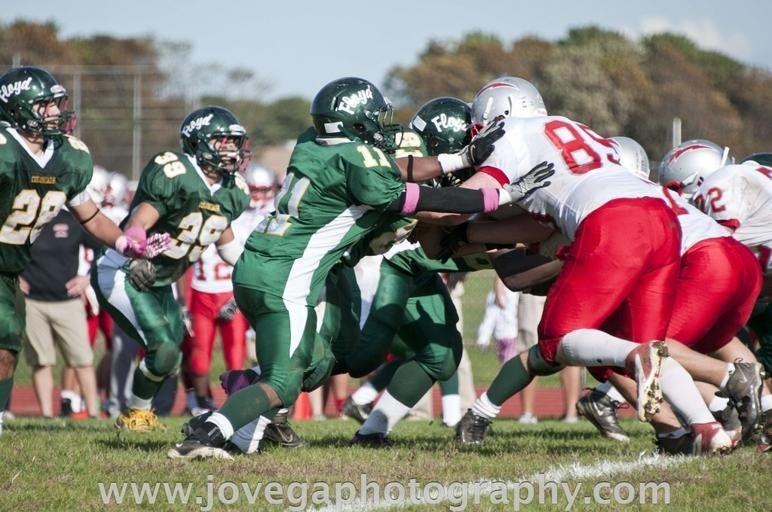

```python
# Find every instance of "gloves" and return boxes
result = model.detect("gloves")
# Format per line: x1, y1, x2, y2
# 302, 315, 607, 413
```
432, 221, 470, 264
128, 256, 158, 293
458, 117, 505, 166
502, 161, 555, 204
115, 231, 175, 260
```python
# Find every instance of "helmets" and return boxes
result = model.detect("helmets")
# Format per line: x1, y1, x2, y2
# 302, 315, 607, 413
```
659, 139, 733, 204
471, 76, 547, 140
86, 165, 108, 203
180, 106, 248, 179
606, 136, 650, 181
103, 172, 128, 205
310, 77, 404, 154
0, 67, 69, 137
242, 163, 278, 211
412, 95, 472, 188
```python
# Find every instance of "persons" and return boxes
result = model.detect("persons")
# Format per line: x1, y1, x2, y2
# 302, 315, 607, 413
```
113, 104, 256, 437
403, 73, 735, 457
219, 92, 770, 457
164, 76, 555, 463
16, 159, 225, 422
0, 64, 176, 443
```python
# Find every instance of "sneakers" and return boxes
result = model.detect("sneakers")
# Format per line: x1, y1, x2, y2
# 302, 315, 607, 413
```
182, 410, 213, 437
576, 387, 630, 445
59, 389, 82, 416
222, 442, 244, 460
348, 430, 405, 450
261, 422, 309, 449
342, 395, 373, 425
561, 416, 579, 424
711, 397, 742, 449
714, 358, 772, 442
516, 414, 538, 424
687, 422, 733, 457
114, 408, 168, 448
165, 438, 234, 464
456, 409, 492, 454
654, 432, 697, 458
